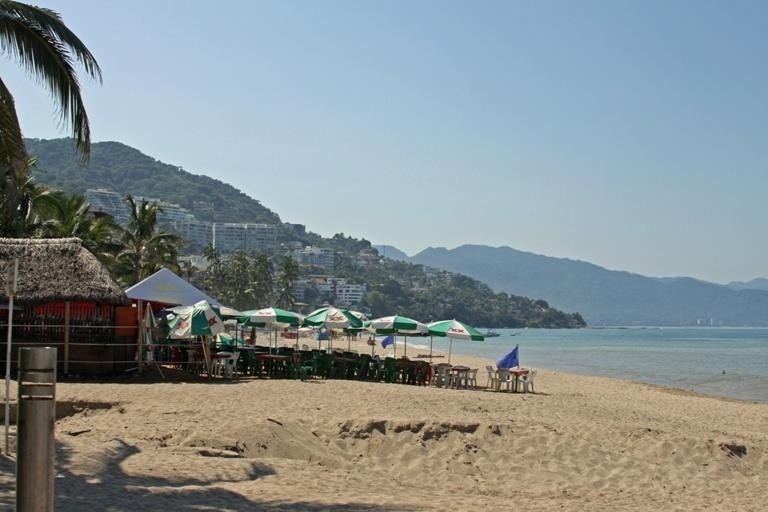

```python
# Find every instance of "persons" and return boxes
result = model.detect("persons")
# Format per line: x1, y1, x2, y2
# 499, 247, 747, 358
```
367, 336, 377, 345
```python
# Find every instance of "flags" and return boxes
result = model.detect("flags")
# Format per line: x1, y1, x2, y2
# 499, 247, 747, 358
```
381, 335, 394, 349
496, 344, 519, 369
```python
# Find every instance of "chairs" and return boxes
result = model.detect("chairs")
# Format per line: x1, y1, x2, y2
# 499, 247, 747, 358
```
485, 365, 536, 392
170, 339, 325, 382
313, 349, 478, 391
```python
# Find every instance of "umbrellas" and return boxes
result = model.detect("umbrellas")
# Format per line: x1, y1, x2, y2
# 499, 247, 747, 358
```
237, 307, 304, 366
156, 299, 225, 378
363, 314, 429, 358
300, 305, 370, 348
211, 304, 249, 324
427, 317, 485, 366
